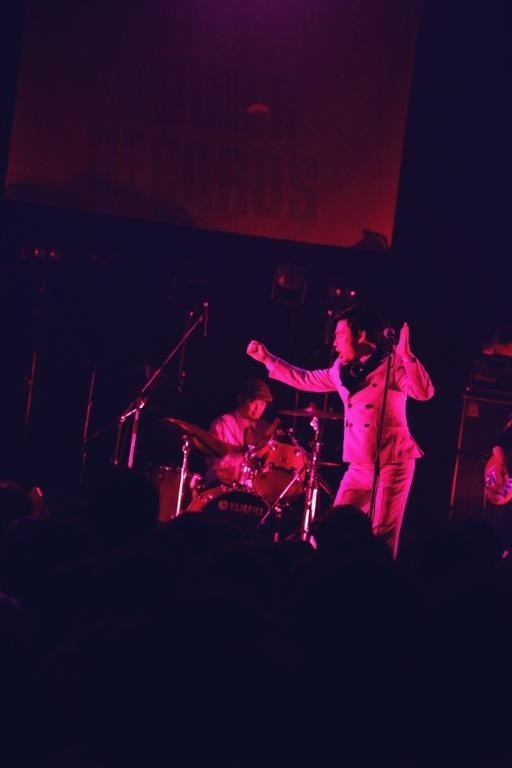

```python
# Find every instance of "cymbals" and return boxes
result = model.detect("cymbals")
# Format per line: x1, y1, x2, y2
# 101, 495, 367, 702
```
165, 417, 226, 458
309, 461, 342, 467
279, 407, 345, 420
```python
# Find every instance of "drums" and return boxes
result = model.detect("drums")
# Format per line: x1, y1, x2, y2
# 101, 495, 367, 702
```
188, 483, 280, 533
156, 466, 196, 521
253, 441, 308, 497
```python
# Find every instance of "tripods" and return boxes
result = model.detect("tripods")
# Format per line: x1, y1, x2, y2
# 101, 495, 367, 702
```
253, 418, 334, 546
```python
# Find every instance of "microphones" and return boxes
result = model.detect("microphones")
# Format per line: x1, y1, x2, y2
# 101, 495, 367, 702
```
203, 299, 208, 339
382, 326, 398, 340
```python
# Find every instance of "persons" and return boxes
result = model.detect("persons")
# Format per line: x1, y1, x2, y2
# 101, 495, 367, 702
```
1, 304, 511, 768
